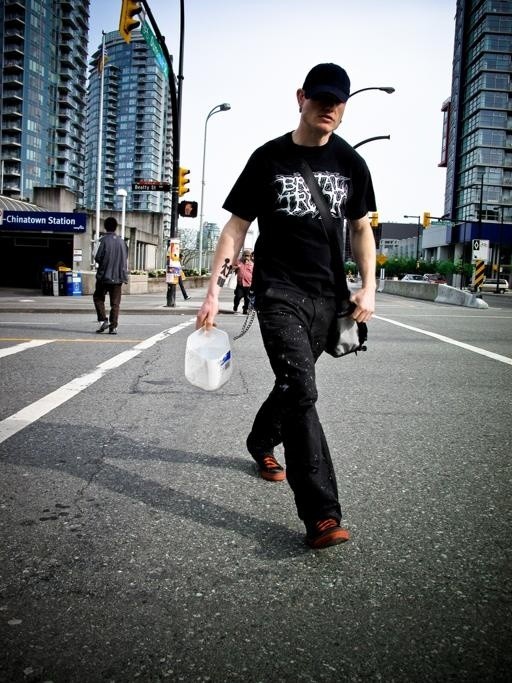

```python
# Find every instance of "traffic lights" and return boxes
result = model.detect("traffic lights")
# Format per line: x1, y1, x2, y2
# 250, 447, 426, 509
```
180, 202, 197, 217
178, 168, 191, 197
118, 1, 142, 43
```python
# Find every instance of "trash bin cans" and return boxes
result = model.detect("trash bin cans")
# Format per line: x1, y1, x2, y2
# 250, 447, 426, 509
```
65, 271, 82, 295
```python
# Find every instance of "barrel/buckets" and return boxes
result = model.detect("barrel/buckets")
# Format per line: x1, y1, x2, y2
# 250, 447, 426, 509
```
185, 327, 232, 388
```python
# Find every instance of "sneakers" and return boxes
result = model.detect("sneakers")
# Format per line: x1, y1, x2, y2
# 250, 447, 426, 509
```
246, 435, 286, 481
186, 297, 192, 300
96, 322, 117, 334
304, 517, 350, 550
233, 306, 249, 314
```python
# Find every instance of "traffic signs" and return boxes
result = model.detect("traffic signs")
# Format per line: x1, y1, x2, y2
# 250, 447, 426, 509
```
132, 183, 169, 192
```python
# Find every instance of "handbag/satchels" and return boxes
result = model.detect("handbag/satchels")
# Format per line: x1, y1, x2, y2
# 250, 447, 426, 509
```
324, 290, 367, 358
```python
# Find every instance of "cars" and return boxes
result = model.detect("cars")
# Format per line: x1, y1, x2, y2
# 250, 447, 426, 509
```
401, 273, 448, 285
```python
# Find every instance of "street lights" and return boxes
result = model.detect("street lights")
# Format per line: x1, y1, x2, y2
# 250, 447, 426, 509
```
349, 87, 395, 97
199, 104, 230, 275
404, 215, 420, 262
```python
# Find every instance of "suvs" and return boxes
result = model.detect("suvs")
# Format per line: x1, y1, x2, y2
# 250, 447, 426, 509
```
469, 279, 510, 294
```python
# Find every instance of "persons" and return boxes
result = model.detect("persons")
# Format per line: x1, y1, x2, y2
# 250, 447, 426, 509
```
195, 62, 377, 549
233, 249, 255, 314
93, 217, 129, 334
179, 254, 191, 300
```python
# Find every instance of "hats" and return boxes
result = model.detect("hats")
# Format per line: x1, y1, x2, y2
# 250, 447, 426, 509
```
242, 250, 251, 255
302, 63, 351, 103
